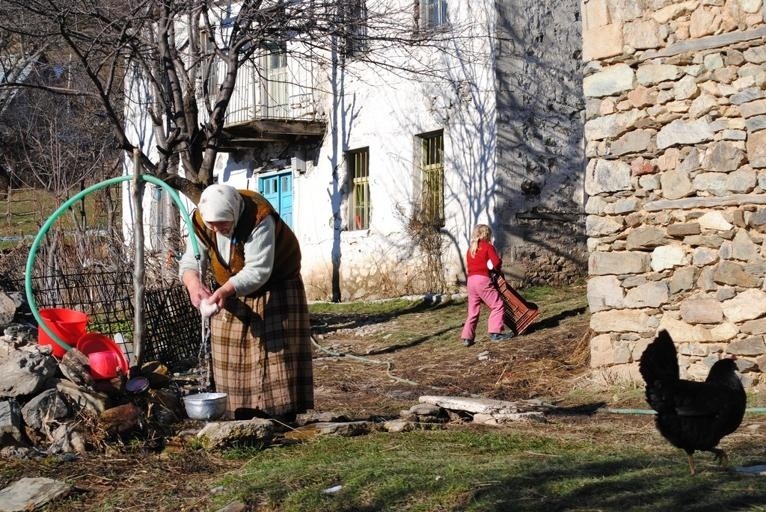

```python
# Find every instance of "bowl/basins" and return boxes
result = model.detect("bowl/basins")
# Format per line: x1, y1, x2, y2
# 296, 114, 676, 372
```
183, 393, 229, 419
77, 332, 129, 380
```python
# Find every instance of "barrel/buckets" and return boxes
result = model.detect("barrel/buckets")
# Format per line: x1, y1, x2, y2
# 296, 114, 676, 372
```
37, 308, 88, 357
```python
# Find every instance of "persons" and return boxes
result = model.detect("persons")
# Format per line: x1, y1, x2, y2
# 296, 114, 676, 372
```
459, 224, 515, 346
177, 181, 315, 422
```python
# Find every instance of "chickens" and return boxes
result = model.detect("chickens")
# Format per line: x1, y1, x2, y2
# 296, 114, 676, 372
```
639, 329, 747, 476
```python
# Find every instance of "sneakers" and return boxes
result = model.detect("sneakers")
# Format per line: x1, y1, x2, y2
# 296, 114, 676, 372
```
490, 333, 513, 341
463, 339, 474, 347
